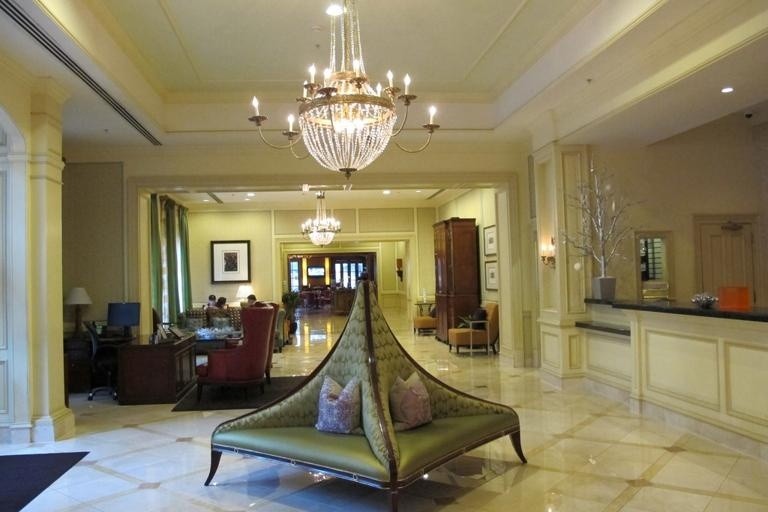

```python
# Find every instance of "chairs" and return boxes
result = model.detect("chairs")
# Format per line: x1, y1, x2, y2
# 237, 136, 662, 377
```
414, 301, 437, 335
83, 321, 117, 399
265, 301, 280, 385
198, 302, 275, 401
449, 303, 499, 353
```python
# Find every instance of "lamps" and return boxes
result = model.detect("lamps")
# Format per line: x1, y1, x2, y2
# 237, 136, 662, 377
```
249, 0, 440, 181
301, 192, 342, 246
538, 244, 556, 266
396, 258, 402, 281
65, 286, 92, 331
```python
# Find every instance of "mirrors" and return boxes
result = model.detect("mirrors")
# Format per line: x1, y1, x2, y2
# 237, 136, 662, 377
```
633, 229, 676, 301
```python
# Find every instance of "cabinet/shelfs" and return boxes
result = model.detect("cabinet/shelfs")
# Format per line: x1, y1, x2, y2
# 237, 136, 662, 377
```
432, 216, 480, 344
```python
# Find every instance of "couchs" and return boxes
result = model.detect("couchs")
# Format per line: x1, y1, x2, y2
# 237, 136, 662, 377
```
204, 272, 528, 512
177, 308, 286, 354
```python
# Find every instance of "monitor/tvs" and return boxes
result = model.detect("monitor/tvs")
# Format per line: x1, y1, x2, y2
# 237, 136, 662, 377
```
307, 266, 325, 277
108, 303, 140, 341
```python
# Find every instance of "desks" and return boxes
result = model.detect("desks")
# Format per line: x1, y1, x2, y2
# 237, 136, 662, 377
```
64, 335, 196, 405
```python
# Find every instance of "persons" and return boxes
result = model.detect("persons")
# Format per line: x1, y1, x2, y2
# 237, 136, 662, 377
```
203, 295, 267, 311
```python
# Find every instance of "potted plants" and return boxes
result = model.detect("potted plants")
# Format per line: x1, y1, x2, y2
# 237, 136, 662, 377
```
282, 293, 305, 333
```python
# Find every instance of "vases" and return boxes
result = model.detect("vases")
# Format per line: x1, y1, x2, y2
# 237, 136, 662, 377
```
591, 276, 617, 299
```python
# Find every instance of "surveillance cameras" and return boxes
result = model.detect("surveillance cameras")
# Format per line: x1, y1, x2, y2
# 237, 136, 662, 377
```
743, 112, 753, 119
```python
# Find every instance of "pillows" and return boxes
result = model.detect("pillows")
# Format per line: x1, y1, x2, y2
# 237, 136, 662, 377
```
316, 376, 364, 434
390, 371, 434, 432
184, 318, 203, 330
211, 318, 230, 328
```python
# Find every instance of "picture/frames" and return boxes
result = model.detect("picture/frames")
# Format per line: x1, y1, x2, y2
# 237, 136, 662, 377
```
484, 260, 499, 291
483, 224, 497, 256
210, 240, 251, 283
